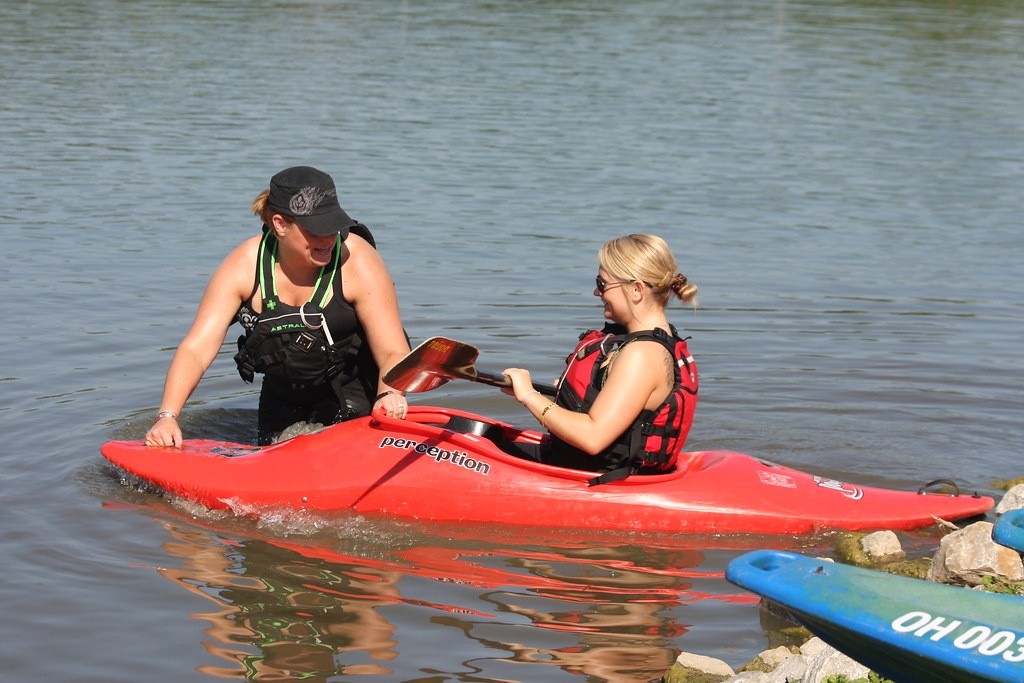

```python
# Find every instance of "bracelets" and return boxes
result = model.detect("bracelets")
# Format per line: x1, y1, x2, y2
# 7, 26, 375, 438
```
541, 403, 558, 426
153, 410, 178, 425
522, 389, 538, 407
374, 391, 394, 402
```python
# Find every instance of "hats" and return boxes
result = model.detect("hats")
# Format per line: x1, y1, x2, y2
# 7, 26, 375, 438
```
267, 165, 358, 238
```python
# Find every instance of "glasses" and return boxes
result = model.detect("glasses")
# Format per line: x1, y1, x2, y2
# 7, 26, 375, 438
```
595, 275, 653, 295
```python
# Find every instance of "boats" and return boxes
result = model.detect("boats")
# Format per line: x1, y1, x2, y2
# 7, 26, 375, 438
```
725, 546, 1024, 683
100, 403, 997, 537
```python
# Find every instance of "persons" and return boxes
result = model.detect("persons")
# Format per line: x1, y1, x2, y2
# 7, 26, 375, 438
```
500, 233, 699, 476
144, 167, 411, 451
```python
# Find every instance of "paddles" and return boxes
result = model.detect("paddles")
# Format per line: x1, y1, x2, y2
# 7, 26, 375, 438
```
382, 337, 559, 395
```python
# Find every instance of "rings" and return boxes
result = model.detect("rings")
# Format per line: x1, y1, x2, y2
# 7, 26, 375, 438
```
399, 404, 404, 407
